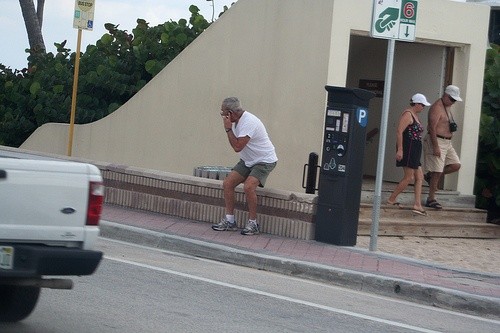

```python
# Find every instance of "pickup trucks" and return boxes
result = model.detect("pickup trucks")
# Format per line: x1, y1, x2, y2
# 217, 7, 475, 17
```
1, 145, 105, 322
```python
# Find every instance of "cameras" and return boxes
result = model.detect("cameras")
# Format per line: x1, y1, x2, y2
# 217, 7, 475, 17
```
450, 123, 457, 132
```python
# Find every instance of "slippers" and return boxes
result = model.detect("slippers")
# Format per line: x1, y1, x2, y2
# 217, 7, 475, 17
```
386, 198, 402, 208
412, 208, 427, 216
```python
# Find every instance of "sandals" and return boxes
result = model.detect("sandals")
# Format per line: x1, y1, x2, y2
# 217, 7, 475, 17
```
424, 171, 438, 191
425, 198, 443, 209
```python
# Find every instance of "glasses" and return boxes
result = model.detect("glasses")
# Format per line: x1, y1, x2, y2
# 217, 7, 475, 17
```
447, 95, 457, 102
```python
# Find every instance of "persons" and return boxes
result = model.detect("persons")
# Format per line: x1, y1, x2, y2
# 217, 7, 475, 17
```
423, 86, 462, 210
212, 98, 279, 236
386, 92, 428, 216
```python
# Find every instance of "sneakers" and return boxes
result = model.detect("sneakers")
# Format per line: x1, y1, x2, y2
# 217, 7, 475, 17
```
211, 218, 238, 231
241, 221, 260, 236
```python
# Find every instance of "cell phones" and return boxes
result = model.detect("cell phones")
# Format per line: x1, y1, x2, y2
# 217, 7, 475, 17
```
226, 110, 233, 118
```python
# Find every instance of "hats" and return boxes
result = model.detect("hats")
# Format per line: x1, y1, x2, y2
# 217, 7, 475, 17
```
445, 85, 462, 102
409, 93, 432, 107
